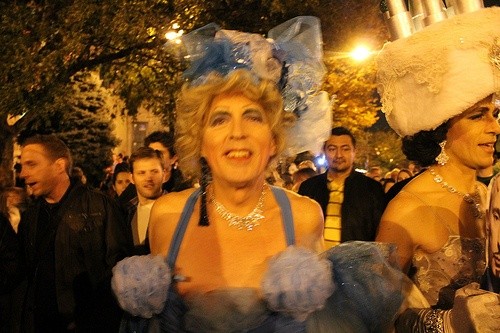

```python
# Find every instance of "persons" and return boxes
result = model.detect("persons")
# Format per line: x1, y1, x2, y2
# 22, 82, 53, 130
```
111, 16, 336, 333
0, 127, 500, 333
375, 6, 500, 333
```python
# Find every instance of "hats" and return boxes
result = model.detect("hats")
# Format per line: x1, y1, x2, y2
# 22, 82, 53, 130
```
373, 0, 499, 137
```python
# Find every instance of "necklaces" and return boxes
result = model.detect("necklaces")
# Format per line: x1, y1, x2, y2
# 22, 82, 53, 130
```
427, 166, 485, 220
203, 180, 266, 231
